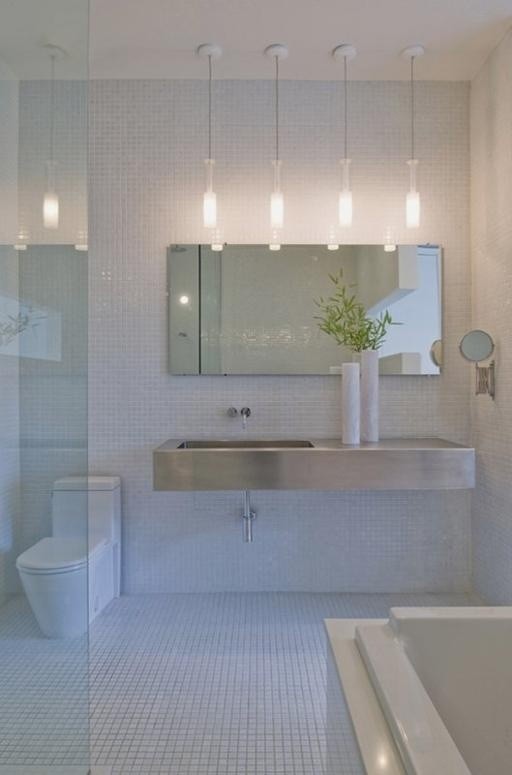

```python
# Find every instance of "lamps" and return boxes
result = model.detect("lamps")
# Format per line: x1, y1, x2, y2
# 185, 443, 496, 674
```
264, 45, 288, 230
39, 44, 69, 230
403, 46, 424, 228
198, 43, 221, 230
333, 45, 356, 229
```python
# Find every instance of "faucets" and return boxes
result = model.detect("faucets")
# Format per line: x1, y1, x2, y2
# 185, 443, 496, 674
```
240, 407, 251, 428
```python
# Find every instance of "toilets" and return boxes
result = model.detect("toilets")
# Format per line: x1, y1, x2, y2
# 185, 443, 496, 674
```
14, 477, 121, 639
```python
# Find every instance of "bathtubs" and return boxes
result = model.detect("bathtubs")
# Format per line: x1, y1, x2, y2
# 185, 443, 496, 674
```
318, 606, 512, 773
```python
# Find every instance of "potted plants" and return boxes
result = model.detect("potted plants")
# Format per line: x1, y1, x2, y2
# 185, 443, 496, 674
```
329, 266, 403, 442
310, 296, 362, 363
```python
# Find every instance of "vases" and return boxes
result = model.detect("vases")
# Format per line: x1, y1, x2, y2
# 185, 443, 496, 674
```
341, 363, 359, 446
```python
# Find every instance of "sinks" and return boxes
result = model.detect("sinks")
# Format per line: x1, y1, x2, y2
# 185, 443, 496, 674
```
176, 439, 315, 449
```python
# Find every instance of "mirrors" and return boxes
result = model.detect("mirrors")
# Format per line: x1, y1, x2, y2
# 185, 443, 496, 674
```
459, 330, 495, 363
430, 339, 442, 366
165, 243, 445, 377
